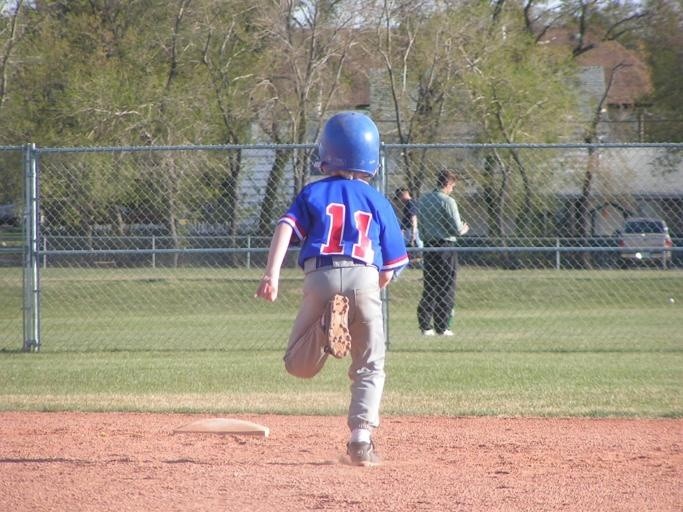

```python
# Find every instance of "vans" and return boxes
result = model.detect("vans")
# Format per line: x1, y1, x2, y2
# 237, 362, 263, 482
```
616, 217, 674, 270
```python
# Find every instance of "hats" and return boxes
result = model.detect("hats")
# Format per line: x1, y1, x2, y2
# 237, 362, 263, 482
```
393, 187, 408, 200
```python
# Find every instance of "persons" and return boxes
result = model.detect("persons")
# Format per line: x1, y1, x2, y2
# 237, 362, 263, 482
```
253, 112, 411, 468
417, 168, 468, 337
392, 186, 423, 269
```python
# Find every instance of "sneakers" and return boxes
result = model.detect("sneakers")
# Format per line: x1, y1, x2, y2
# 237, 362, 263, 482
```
324, 294, 352, 358
443, 329, 455, 335
347, 438, 380, 465
420, 326, 435, 336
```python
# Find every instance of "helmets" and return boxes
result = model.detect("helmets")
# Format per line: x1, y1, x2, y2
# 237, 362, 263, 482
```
318, 112, 380, 176
